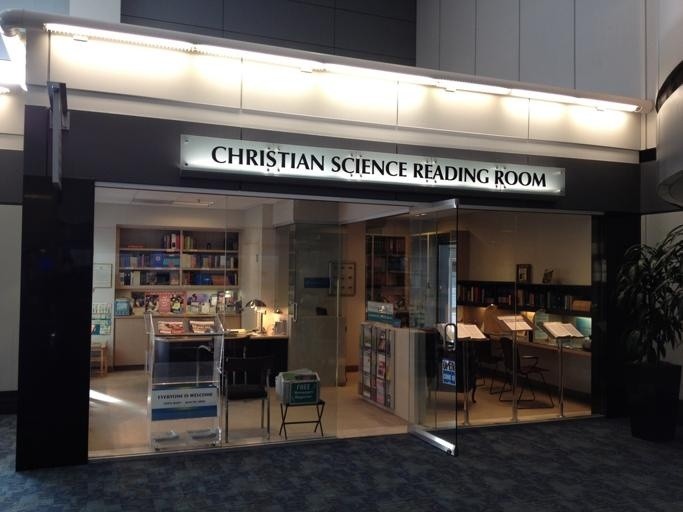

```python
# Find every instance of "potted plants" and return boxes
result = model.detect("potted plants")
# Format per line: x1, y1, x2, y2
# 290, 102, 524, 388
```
610, 222, 683, 444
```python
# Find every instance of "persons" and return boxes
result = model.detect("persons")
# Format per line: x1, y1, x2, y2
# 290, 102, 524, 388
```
146, 296, 160, 313
171, 296, 184, 313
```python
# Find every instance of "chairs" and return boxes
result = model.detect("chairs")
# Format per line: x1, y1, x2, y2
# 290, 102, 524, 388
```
493, 335, 557, 411
468, 331, 514, 396
218, 352, 272, 444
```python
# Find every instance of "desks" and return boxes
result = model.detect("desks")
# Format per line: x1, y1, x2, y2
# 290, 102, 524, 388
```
147, 333, 287, 401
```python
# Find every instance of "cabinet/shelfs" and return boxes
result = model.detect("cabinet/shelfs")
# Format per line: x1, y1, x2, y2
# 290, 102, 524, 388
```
112, 224, 242, 370
452, 280, 590, 318
358, 228, 473, 330
421, 325, 480, 404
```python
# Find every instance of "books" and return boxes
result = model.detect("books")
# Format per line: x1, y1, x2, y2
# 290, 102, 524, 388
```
436, 323, 486, 342
361, 326, 391, 406
497, 315, 533, 331
120, 234, 238, 286
189, 320, 214, 326
543, 320, 584, 338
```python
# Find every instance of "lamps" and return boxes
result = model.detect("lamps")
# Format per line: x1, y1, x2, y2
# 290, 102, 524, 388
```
240, 297, 268, 335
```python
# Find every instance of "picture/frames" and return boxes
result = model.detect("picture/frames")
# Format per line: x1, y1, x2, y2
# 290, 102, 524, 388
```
515, 263, 532, 284
325, 259, 357, 298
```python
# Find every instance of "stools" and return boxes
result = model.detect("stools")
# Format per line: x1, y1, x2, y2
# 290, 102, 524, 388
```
278, 400, 326, 440
90, 340, 109, 376
